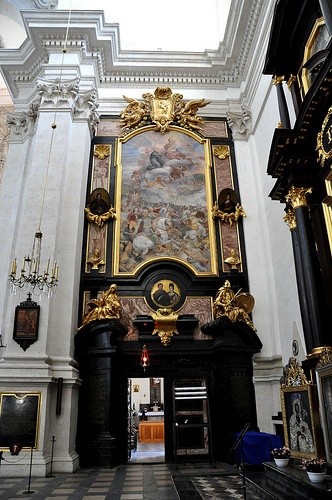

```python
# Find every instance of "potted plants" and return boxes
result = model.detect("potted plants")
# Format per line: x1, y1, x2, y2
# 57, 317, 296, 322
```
270, 446, 291, 467
301, 457, 327, 483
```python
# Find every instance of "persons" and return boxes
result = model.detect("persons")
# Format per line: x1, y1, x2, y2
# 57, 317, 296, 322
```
121, 103, 146, 129
215, 279, 258, 329
100, 206, 118, 229
85, 204, 99, 229
88, 247, 106, 270
154, 117, 174, 135
230, 204, 247, 228
175, 103, 205, 130
159, 101, 170, 116
167, 283, 180, 306
154, 284, 170, 307
212, 201, 230, 224
84, 284, 127, 325
225, 248, 244, 271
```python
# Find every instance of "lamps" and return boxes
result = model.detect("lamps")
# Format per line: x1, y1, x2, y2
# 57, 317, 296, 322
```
7, 0, 75, 303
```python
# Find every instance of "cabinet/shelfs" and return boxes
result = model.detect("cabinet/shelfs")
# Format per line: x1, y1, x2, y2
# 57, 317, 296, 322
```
138, 421, 165, 443
262, 461, 332, 500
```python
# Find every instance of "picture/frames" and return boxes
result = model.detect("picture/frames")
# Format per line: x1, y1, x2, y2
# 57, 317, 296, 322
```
315, 363, 332, 465
279, 384, 316, 459
